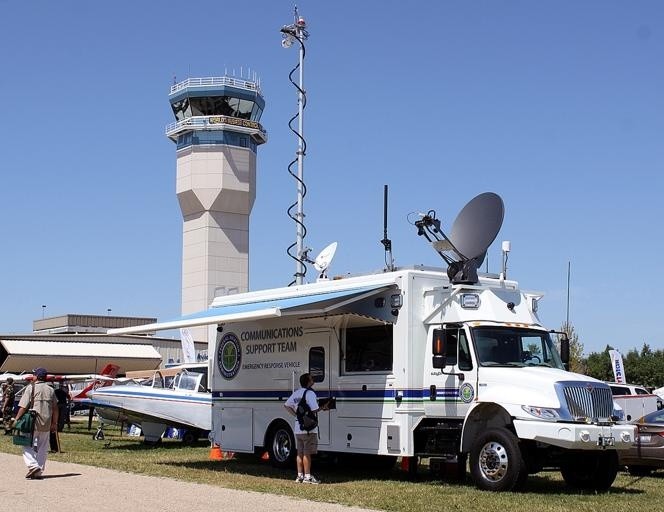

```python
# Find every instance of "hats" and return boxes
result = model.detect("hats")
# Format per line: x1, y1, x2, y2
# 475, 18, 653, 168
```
33, 367, 47, 377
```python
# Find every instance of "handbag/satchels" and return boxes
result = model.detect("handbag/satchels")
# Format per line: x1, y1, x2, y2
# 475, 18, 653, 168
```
12, 409, 36, 447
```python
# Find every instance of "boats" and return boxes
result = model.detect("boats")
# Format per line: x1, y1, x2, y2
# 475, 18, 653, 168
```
85, 368, 211, 446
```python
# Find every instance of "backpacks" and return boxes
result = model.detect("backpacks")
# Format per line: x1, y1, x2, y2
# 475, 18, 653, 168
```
295, 388, 319, 431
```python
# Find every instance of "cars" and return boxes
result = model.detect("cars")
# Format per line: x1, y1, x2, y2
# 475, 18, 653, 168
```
604, 382, 664, 476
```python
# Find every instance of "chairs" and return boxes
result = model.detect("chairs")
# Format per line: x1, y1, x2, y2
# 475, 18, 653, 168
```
490, 335, 520, 364
444, 334, 469, 363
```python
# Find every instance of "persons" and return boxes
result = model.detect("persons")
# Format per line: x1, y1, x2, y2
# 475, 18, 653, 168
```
284, 373, 329, 484
2, 368, 71, 479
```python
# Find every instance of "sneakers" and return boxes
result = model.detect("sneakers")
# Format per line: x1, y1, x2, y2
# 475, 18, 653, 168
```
25, 466, 39, 478
295, 476, 321, 485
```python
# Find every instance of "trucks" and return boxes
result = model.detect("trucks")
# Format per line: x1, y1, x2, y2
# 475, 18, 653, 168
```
107, 5, 637, 493
